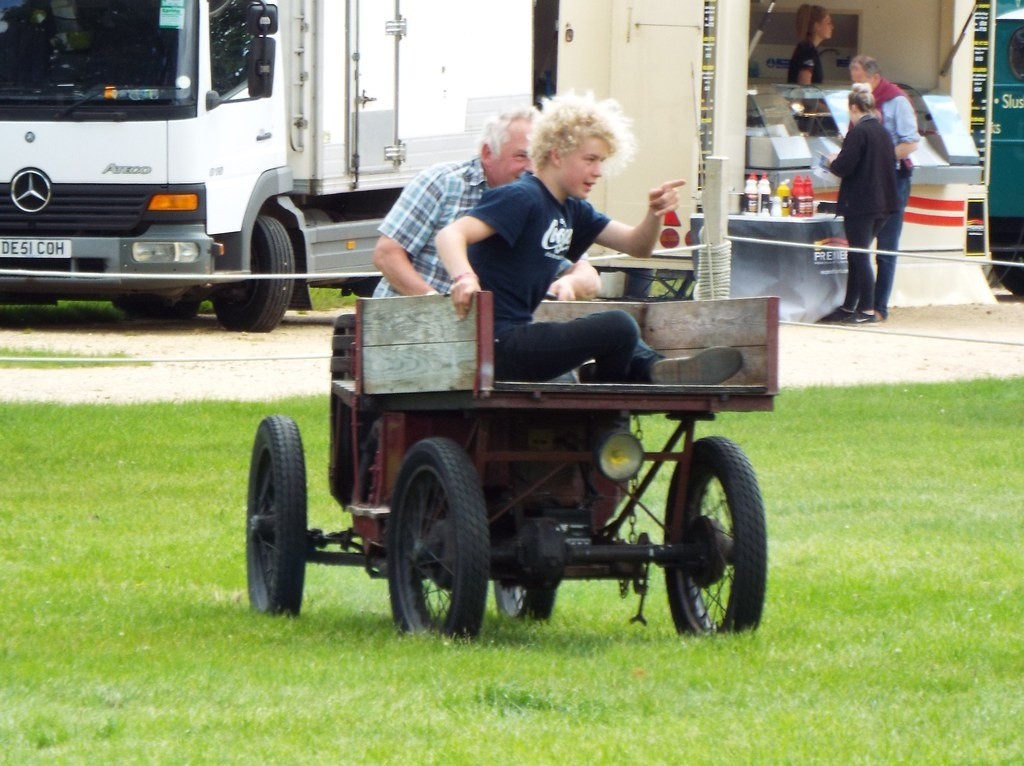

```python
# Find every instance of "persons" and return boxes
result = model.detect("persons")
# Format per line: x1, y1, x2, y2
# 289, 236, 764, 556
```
435, 88, 744, 385
818, 82, 898, 325
371, 103, 604, 303
848, 54, 920, 322
788, 4, 834, 131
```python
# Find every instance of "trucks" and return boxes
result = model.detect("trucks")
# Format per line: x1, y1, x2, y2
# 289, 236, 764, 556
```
557, 0, 1024, 321
0, 0, 534, 331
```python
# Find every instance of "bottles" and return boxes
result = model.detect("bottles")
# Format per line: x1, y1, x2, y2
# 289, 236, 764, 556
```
746, 172, 814, 218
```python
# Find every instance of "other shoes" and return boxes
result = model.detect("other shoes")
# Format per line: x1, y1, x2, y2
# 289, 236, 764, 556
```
873, 308, 885, 322
648, 342, 745, 386
578, 358, 601, 384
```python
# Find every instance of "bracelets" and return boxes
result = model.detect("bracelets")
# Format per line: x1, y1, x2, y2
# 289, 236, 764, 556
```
427, 290, 439, 295
455, 273, 478, 282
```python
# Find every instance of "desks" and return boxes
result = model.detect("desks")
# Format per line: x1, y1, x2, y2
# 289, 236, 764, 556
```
691, 215, 874, 324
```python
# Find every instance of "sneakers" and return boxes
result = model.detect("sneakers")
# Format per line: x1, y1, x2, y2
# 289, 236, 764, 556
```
840, 308, 876, 325
815, 306, 855, 323
